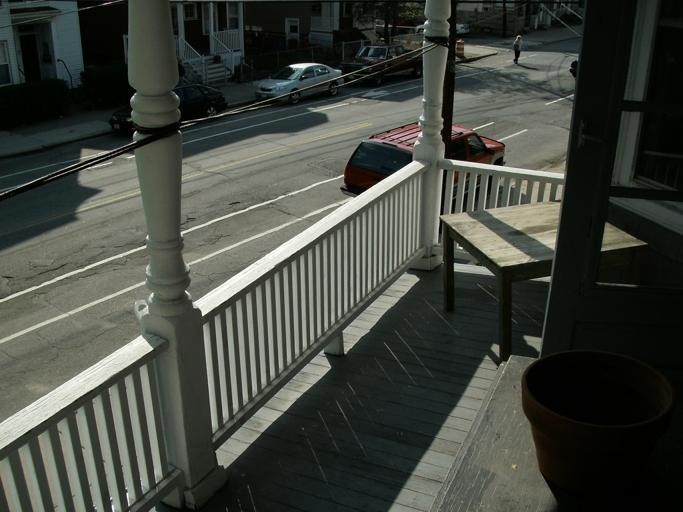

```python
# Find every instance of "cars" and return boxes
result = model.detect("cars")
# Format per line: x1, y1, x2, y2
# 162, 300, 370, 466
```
375, 19, 399, 39
109, 84, 228, 134
255, 45, 422, 104
415, 22, 470, 34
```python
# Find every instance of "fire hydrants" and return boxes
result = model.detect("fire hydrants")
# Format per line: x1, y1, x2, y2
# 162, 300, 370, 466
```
456, 39, 464, 57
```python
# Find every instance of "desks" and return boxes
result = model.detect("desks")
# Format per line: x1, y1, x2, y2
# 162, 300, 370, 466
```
437, 199, 646, 364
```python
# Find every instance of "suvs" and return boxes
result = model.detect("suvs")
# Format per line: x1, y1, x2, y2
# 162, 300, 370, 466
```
340, 119, 506, 217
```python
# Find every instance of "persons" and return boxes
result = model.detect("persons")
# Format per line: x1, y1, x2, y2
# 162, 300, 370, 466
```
513, 35, 522, 63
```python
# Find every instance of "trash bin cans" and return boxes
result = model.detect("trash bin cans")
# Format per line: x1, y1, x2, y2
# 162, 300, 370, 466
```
530, 16, 538, 30
545, 14, 551, 25
